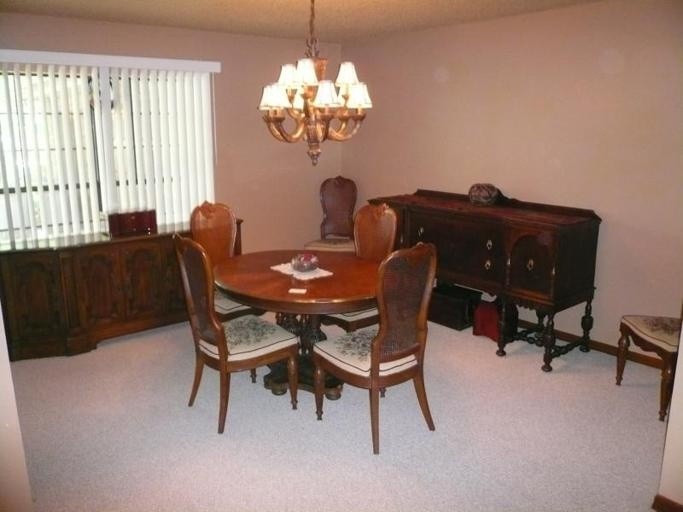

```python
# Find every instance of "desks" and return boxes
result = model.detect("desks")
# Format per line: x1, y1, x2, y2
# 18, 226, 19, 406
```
208, 245, 383, 402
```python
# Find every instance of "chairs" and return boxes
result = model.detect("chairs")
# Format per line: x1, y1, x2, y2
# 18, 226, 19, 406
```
301, 173, 361, 255
168, 230, 299, 435
183, 199, 270, 386
319, 195, 398, 341
309, 235, 437, 455
611, 293, 682, 423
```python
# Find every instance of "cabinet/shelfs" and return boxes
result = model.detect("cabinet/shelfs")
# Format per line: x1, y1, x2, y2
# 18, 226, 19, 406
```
371, 187, 602, 374
0, 214, 245, 364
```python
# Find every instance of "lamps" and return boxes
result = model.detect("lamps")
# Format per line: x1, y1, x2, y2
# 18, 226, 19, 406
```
258, 0, 374, 170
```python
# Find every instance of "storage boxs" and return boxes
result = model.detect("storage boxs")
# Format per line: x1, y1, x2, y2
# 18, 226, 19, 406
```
425, 280, 480, 333
100, 204, 162, 237
472, 300, 522, 343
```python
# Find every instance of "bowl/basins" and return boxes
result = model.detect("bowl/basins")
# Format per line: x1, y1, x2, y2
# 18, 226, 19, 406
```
290, 254, 319, 272
